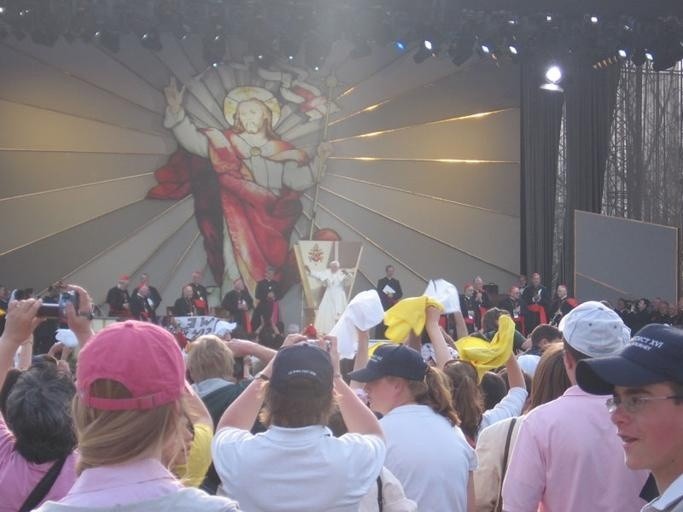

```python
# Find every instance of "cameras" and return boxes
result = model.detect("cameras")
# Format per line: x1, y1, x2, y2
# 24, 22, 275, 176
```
35, 289, 79, 319
299, 340, 331, 353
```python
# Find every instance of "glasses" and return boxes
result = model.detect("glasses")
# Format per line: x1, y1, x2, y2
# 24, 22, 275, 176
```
605, 396, 678, 411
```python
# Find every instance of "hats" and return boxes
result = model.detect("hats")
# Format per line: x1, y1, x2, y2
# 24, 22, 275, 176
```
558, 301, 625, 356
270, 344, 334, 389
347, 343, 423, 383
575, 324, 682, 394
74, 321, 187, 410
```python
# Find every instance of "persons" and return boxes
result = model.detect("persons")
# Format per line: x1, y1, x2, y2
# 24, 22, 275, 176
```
0, 260, 682, 512
163, 75, 333, 302
